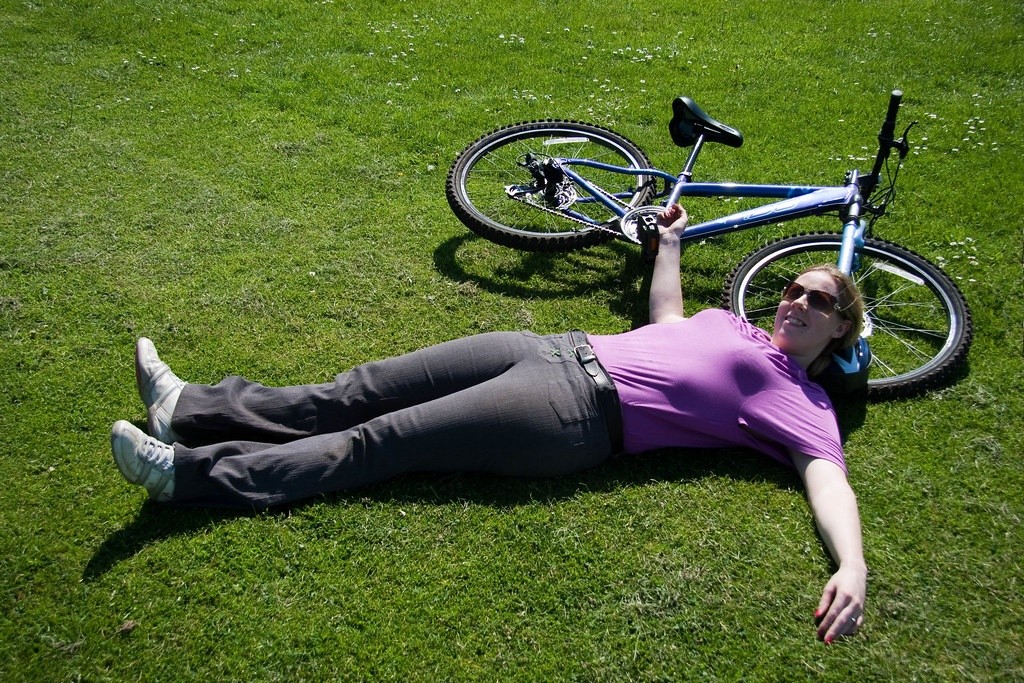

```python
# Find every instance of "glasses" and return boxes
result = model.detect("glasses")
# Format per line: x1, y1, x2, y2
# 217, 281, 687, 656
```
780, 282, 847, 321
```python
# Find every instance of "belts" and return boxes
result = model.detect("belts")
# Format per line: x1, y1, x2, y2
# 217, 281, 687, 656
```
570, 330, 624, 461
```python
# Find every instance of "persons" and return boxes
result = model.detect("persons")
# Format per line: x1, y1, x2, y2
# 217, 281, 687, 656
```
111, 199, 877, 646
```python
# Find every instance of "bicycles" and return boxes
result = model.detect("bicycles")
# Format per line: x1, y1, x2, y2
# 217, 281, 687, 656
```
440, 81, 971, 403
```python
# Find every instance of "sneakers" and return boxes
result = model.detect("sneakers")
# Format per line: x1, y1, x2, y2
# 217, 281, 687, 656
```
135, 336, 189, 444
111, 420, 177, 504
